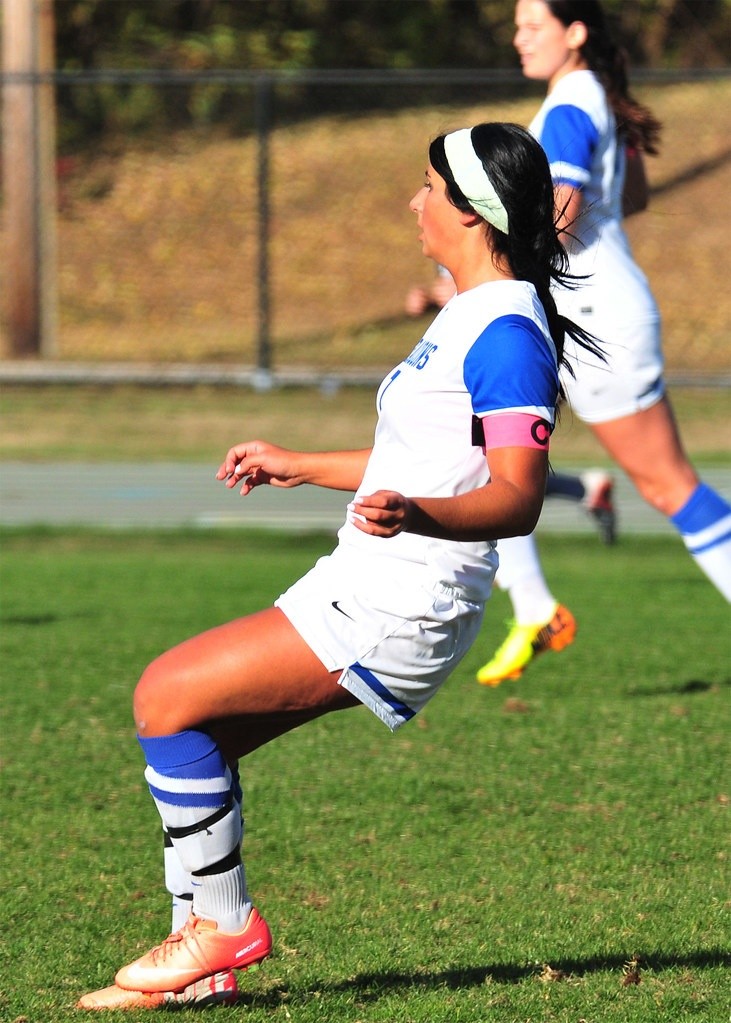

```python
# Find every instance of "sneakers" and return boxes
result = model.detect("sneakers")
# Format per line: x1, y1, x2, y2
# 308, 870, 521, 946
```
475, 602, 576, 686
581, 467, 616, 546
77, 969, 237, 1011
114, 907, 271, 996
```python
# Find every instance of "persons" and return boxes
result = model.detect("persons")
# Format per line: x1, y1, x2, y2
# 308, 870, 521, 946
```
534, 458, 624, 550
400, 1, 731, 689
65, 116, 571, 1012
